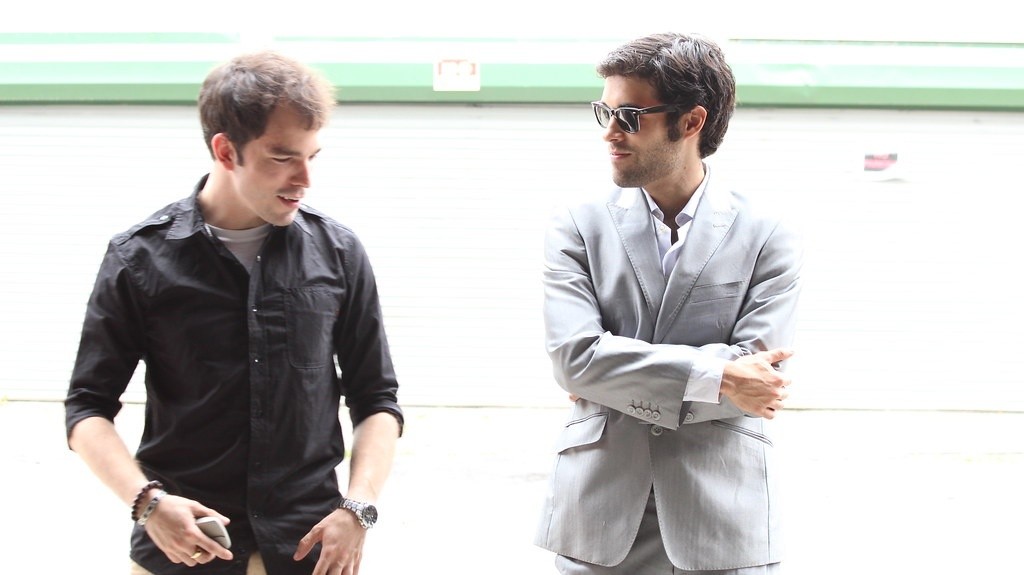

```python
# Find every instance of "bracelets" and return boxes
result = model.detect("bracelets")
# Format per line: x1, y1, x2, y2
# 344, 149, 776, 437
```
131, 480, 167, 526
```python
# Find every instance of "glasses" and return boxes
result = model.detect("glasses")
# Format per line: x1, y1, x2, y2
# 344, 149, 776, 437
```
591, 101, 680, 134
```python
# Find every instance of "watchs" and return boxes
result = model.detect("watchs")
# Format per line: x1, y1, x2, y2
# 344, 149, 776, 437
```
338, 498, 378, 529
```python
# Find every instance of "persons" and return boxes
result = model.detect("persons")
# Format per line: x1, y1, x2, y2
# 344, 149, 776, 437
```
534, 33, 802, 575
65, 51, 403, 575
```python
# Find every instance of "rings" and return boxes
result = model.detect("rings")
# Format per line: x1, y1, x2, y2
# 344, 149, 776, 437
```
190, 548, 202, 559
